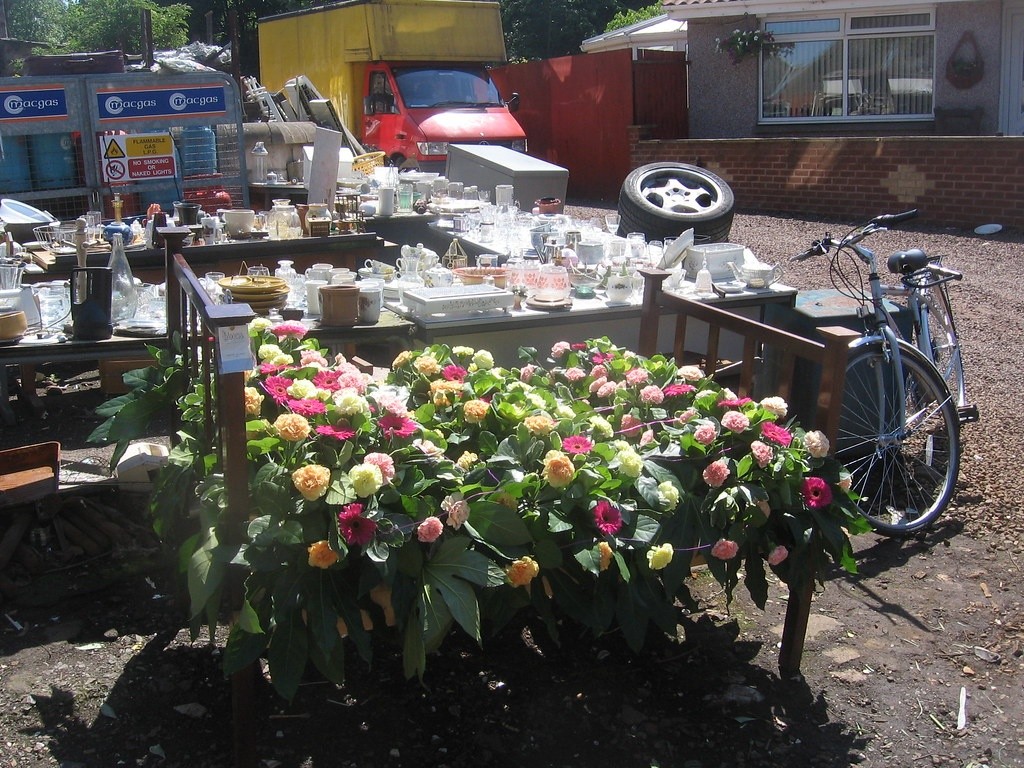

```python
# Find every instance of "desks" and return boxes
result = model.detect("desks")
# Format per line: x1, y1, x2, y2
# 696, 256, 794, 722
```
0, 178, 914, 462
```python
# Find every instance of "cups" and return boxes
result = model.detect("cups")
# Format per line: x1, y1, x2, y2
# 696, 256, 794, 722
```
0, 164, 787, 341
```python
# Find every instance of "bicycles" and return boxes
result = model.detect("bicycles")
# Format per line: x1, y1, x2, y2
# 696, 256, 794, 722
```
787, 208, 979, 538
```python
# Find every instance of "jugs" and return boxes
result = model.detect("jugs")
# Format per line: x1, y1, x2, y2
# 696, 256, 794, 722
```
727, 261, 785, 289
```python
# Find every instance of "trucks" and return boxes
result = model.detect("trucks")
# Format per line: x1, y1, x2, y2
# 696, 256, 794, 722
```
258, 0, 529, 180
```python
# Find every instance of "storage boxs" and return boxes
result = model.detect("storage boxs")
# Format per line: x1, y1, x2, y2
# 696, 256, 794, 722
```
444, 143, 569, 215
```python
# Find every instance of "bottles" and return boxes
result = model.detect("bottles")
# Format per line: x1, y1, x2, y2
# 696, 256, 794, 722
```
251, 142, 268, 185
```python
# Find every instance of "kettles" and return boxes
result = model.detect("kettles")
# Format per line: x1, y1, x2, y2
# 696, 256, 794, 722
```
68, 266, 113, 341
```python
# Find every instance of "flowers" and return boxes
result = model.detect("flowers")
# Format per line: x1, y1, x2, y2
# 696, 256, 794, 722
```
88, 319, 873, 711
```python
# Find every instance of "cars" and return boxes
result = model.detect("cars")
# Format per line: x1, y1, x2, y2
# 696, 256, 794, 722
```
762, 90, 902, 117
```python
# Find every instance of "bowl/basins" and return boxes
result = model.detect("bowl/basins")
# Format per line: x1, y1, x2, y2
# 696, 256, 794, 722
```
0, 198, 51, 223
3, 222, 51, 245
